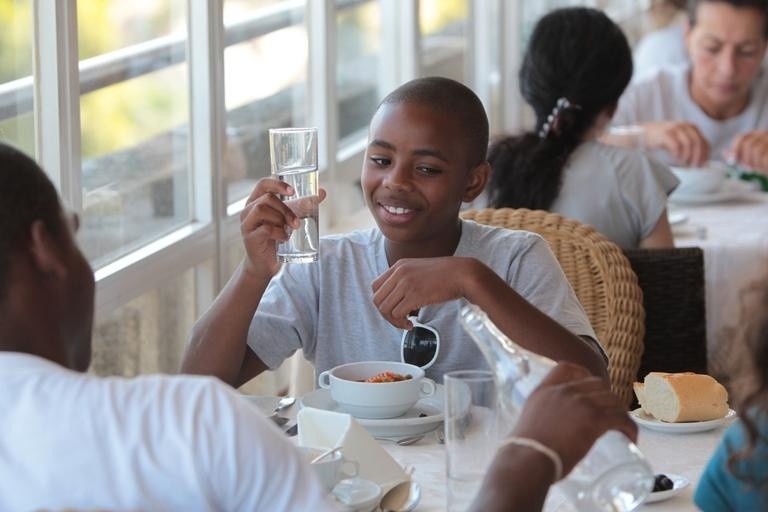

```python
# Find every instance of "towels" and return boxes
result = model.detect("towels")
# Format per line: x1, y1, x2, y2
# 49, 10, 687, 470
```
297, 405, 409, 511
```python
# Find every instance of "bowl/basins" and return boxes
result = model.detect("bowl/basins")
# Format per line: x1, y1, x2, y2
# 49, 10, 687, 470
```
319, 359, 442, 414
672, 164, 736, 189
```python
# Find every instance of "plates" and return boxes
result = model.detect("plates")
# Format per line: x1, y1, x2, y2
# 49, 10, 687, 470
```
641, 469, 691, 503
242, 393, 300, 433
667, 204, 690, 224
667, 181, 752, 206
623, 404, 742, 433
303, 380, 442, 444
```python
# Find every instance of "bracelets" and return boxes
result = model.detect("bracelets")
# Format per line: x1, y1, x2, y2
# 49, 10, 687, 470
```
495, 436, 565, 480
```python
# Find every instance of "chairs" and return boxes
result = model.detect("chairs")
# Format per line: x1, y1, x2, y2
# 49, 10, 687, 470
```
623, 246, 705, 382
458, 206, 648, 412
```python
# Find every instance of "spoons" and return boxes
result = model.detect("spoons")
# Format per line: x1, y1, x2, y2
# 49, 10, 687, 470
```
382, 482, 421, 512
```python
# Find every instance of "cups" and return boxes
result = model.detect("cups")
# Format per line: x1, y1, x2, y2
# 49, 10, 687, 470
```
442, 368, 500, 498
268, 126, 321, 266
610, 124, 648, 154
295, 447, 361, 486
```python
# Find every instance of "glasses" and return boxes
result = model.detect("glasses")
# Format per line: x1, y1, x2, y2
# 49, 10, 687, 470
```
400, 316, 441, 368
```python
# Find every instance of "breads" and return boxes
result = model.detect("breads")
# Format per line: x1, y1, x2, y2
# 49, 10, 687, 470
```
631, 372, 730, 424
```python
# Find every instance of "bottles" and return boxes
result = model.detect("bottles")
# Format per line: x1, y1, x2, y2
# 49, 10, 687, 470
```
461, 305, 657, 512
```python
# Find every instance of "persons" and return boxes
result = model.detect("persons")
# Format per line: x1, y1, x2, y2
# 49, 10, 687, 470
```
473, 8, 680, 262
629, 1, 687, 65
176, 75, 610, 408
599, 0, 768, 181
1, 141, 638, 512
693, 248, 768, 511
